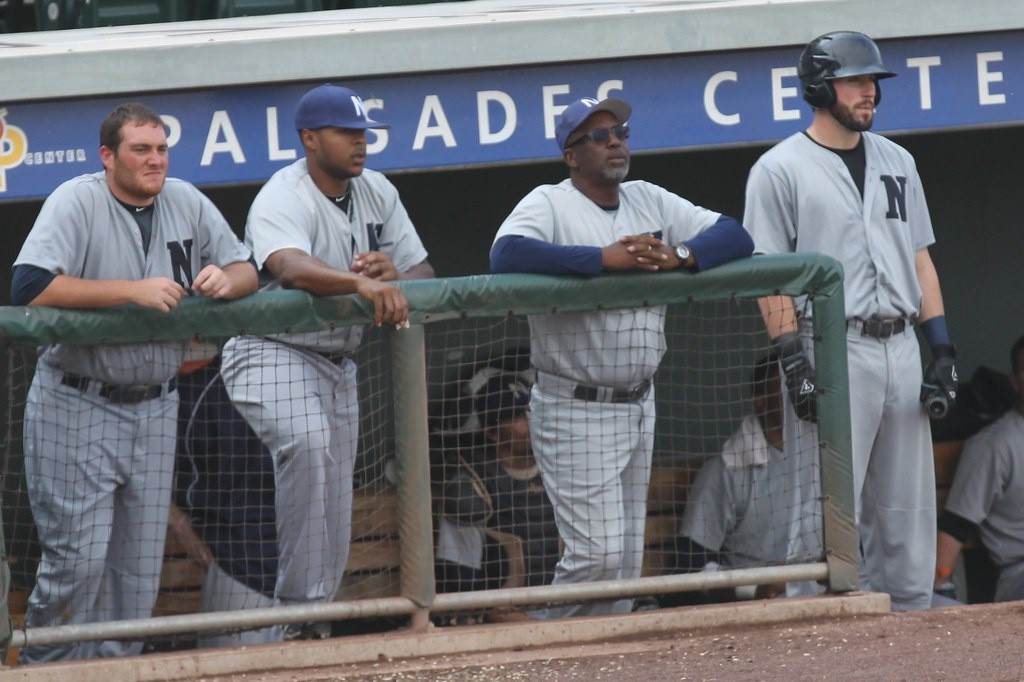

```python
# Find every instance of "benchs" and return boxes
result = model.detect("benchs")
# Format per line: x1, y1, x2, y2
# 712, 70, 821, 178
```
0, 440, 965, 668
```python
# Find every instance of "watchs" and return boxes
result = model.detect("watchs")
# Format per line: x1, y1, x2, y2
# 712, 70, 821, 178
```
674, 241, 690, 269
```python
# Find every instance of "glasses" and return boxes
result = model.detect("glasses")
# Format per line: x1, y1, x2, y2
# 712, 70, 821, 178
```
564, 126, 630, 149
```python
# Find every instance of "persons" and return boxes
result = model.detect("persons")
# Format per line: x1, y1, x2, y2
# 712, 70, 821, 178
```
488, 93, 755, 620
166, 336, 285, 650
8, 102, 260, 666
669, 349, 967, 608
743, 31, 959, 611
436, 349, 662, 623
218, 81, 434, 639
934, 337, 1024, 601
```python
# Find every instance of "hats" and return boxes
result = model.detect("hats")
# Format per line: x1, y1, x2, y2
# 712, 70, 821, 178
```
474, 375, 533, 425
555, 97, 631, 151
295, 83, 392, 130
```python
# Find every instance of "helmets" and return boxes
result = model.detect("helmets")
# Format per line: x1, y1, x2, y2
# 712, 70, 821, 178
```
797, 31, 898, 110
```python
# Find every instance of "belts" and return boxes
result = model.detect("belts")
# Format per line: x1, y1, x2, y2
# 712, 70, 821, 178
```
61, 373, 177, 404
265, 337, 344, 365
846, 312, 918, 339
535, 370, 650, 403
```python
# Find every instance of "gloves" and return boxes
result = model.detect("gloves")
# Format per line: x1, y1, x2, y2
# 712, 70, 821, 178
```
781, 356, 817, 423
920, 344, 960, 409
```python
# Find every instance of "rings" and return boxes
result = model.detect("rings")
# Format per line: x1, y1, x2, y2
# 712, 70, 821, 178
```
648, 245, 651, 250
